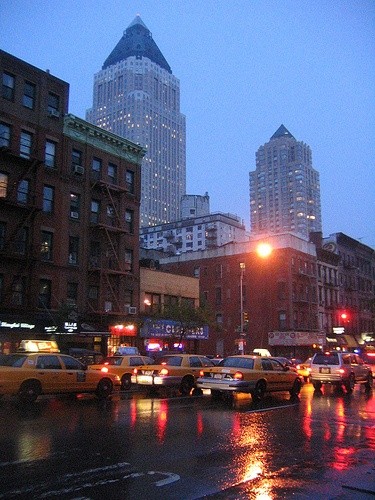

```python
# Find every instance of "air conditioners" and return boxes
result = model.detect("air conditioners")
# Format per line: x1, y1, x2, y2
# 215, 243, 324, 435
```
319, 301, 325, 305
72, 165, 84, 175
70, 211, 78, 219
126, 307, 137, 314
48, 109, 60, 118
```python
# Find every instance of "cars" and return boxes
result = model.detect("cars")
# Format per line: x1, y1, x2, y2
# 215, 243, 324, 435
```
362, 352, 375, 379
0, 338, 122, 406
209, 357, 224, 365
69, 345, 156, 389
295, 357, 312, 383
196, 348, 305, 404
130, 354, 217, 396
271, 356, 295, 367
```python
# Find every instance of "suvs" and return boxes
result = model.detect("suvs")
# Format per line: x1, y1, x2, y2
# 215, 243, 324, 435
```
309, 350, 373, 392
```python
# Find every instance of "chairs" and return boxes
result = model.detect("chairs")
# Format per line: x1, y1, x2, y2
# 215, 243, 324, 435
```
327, 358, 336, 365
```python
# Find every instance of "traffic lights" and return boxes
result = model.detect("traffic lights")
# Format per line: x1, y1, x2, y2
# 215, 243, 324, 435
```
243, 312, 248, 323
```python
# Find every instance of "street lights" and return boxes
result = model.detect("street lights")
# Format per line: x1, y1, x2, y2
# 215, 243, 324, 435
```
240, 243, 270, 355
